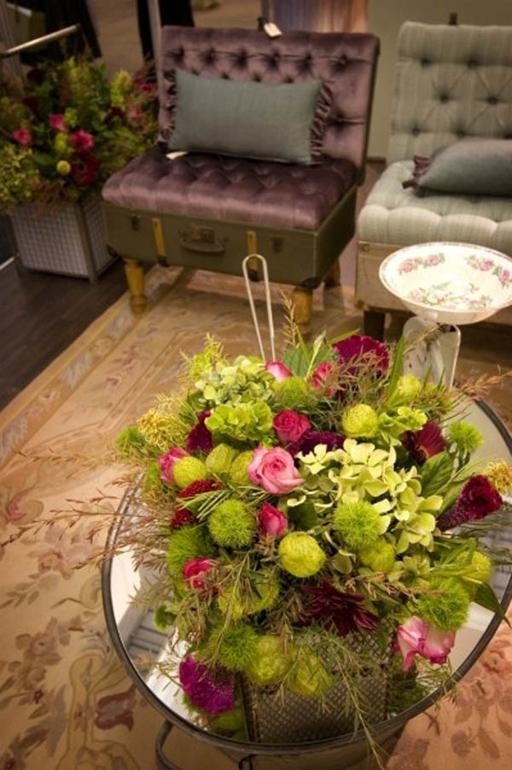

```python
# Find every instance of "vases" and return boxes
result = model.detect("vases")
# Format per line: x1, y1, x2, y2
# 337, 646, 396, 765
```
234, 627, 389, 744
8, 189, 118, 283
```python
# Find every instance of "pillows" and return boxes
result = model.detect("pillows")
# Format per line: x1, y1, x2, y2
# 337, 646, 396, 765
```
168, 66, 332, 168
413, 138, 512, 199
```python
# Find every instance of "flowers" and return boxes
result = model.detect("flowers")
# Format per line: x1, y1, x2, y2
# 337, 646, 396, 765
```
0, 54, 160, 204
117, 329, 512, 770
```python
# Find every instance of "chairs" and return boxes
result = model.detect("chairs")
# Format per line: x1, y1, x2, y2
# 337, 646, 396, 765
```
101, 24, 380, 342
352, 19, 511, 343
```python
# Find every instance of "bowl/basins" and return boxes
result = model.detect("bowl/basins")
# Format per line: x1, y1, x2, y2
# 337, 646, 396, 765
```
377, 240, 512, 328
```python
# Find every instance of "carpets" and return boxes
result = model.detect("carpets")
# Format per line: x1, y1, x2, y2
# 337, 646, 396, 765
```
1, 262, 512, 770
